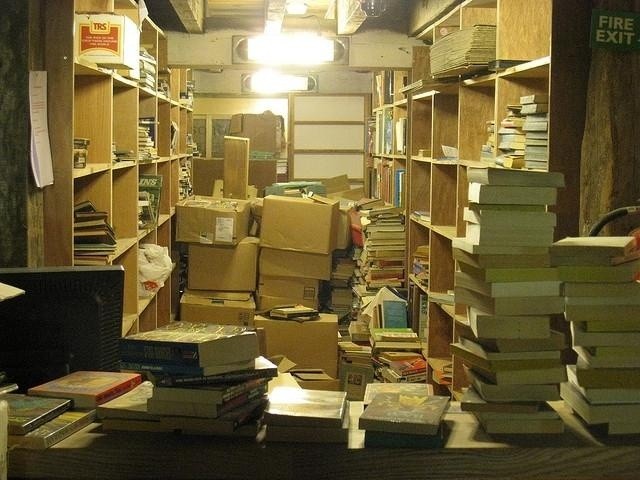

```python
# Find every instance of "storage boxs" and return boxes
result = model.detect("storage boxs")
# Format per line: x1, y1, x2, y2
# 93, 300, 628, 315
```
174, 194, 350, 392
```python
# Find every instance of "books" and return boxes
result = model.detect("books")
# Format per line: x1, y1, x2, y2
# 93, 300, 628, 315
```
70, 2, 196, 165
347, 24, 550, 165
1, 164, 640, 452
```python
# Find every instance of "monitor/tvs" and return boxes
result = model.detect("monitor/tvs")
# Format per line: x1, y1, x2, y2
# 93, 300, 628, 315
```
0, 265, 125, 387
